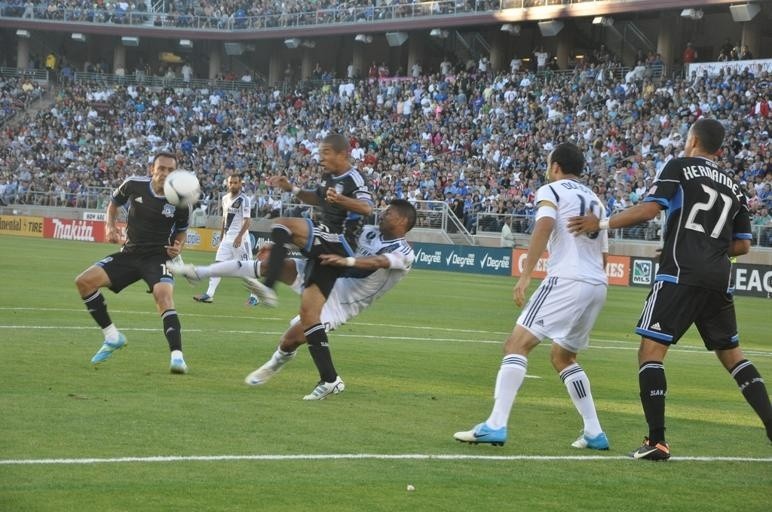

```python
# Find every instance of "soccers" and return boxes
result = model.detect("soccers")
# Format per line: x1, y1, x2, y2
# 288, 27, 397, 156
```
163, 169, 203, 207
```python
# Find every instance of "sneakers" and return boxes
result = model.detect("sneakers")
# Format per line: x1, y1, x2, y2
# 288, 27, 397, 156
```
302, 373, 346, 402
244, 357, 282, 386
192, 292, 213, 303
570, 432, 610, 451
91, 332, 127, 365
166, 260, 202, 289
170, 358, 186, 373
243, 274, 277, 310
628, 436, 671, 462
245, 295, 259, 306
452, 422, 508, 449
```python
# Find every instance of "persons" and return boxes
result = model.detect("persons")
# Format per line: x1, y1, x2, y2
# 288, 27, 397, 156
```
192, 172, 263, 309
164, 197, 418, 388
241, 133, 377, 403
71, 151, 191, 376
565, 117, 772, 461
451, 140, 614, 454
1, 0, 772, 254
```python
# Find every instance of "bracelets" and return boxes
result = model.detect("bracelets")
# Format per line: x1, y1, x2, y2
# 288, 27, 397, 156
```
598, 215, 611, 230
291, 186, 301, 195
345, 255, 356, 268
